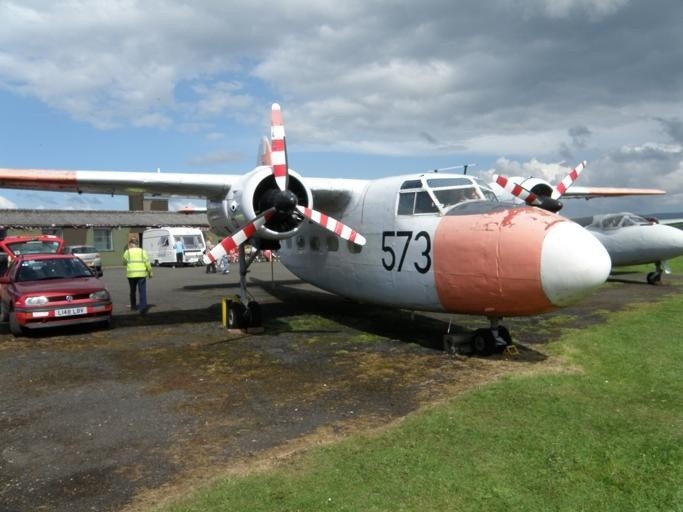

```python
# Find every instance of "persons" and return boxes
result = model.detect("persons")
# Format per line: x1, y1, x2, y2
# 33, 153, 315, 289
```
218, 240, 230, 273
174, 237, 185, 267
122, 238, 153, 314
206, 236, 216, 274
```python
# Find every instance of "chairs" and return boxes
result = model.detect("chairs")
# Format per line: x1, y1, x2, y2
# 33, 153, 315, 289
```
18, 264, 59, 282
